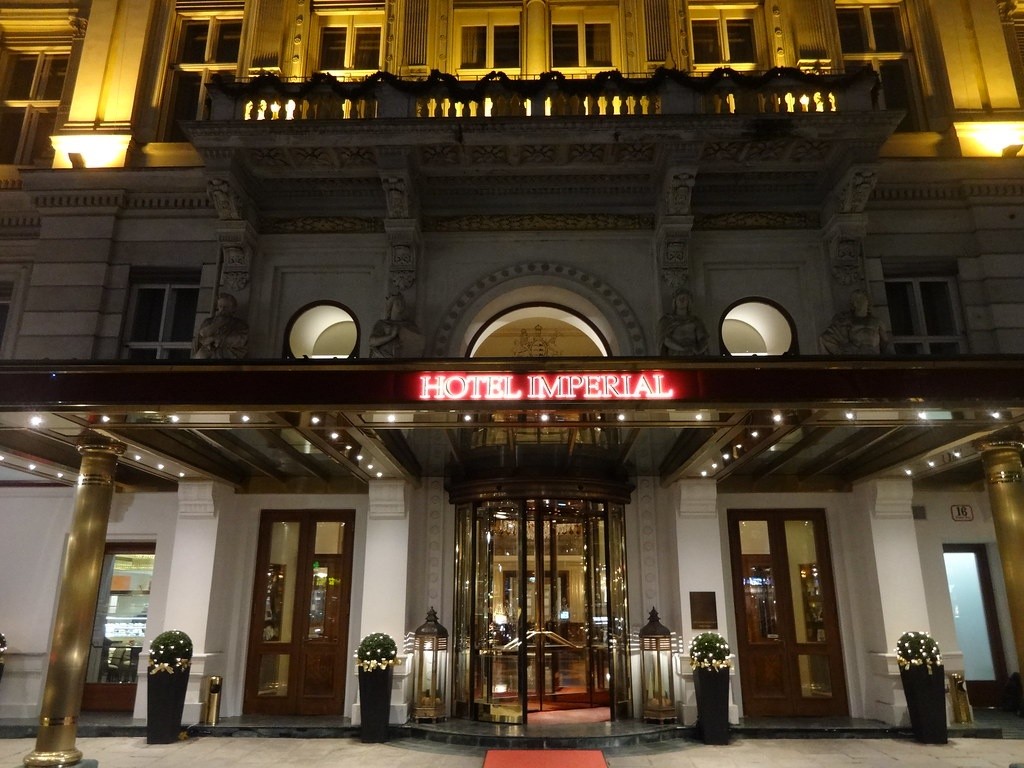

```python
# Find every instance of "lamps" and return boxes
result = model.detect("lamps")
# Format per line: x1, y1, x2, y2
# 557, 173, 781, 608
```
410, 604, 448, 723
639, 606, 679, 726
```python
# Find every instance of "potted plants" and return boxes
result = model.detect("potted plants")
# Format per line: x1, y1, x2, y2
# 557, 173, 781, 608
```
689, 630, 737, 746
356, 633, 402, 743
146, 630, 193, 745
895, 631, 948, 744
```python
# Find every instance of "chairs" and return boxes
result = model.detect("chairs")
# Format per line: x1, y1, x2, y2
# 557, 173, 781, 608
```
101, 647, 125, 681
123, 646, 143, 682
99, 647, 120, 682
